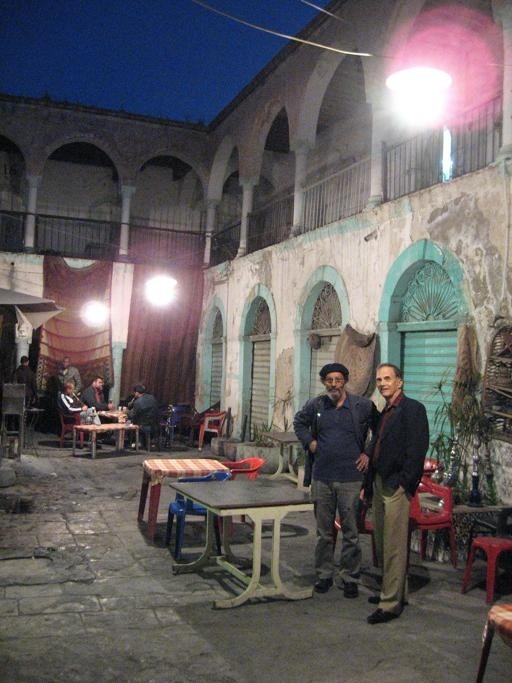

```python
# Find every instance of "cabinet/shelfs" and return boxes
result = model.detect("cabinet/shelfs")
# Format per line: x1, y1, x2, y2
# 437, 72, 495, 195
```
478, 323, 512, 446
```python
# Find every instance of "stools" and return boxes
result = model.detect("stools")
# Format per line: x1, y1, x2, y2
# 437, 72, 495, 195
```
461, 536, 512, 607
475, 595, 511, 683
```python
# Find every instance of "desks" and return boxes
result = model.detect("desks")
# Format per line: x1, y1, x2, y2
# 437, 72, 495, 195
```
262, 429, 302, 486
169, 480, 321, 610
136, 457, 237, 552
24, 407, 47, 458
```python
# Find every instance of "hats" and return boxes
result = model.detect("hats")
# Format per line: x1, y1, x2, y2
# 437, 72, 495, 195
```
320, 363, 348, 379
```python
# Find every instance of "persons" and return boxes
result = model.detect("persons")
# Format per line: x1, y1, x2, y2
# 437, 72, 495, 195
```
358, 363, 430, 624
12, 355, 37, 402
57, 355, 82, 395
126, 384, 162, 448
293, 361, 383, 598
81, 376, 115, 411
56, 382, 101, 427
122, 384, 146, 410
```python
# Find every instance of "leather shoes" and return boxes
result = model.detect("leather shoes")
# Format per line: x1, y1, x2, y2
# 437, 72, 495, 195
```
344, 582, 358, 598
368, 596, 380, 604
315, 578, 332, 593
367, 609, 397, 624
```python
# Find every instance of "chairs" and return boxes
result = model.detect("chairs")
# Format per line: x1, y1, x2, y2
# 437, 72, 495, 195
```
58, 393, 239, 463
333, 457, 459, 570
214, 457, 268, 536
165, 467, 235, 564
